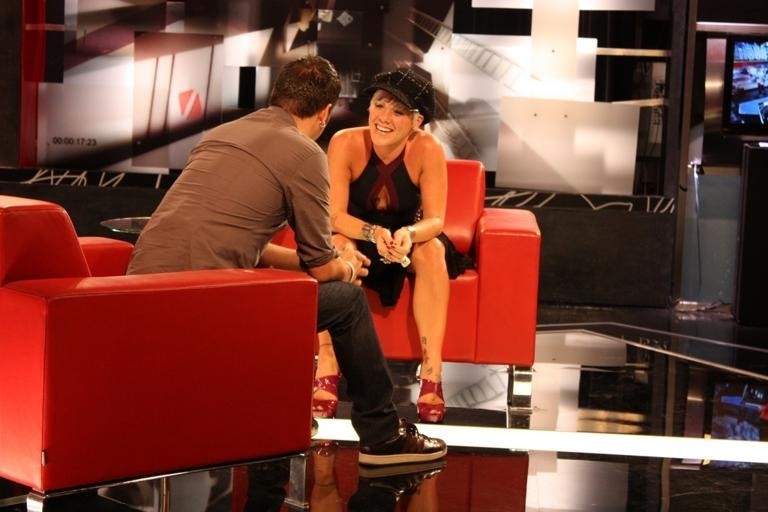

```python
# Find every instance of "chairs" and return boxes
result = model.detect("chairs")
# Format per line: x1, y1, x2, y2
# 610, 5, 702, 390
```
267, 158, 543, 413
0, 193, 320, 512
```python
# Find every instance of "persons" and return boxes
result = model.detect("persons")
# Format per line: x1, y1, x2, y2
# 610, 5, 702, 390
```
204, 458, 448, 512
126, 55, 448, 468
310, 65, 448, 425
309, 442, 438, 512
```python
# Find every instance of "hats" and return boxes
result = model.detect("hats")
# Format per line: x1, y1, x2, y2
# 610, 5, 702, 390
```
362, 69, 434, 125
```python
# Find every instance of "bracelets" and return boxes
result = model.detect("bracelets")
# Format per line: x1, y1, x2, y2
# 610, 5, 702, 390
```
405, 225, 417, 242
362, 222, 381, 244
343, 260, 357, 283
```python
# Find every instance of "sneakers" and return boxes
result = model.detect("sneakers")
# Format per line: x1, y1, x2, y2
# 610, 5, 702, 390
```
357, 417, 446, 467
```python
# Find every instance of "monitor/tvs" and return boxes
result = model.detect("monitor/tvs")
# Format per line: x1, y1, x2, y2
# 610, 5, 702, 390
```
725, 34, 768, 142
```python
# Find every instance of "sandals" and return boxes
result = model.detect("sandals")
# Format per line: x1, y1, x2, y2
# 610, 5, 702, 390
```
313, 370, 342, 419
415, 378, 446, 424
